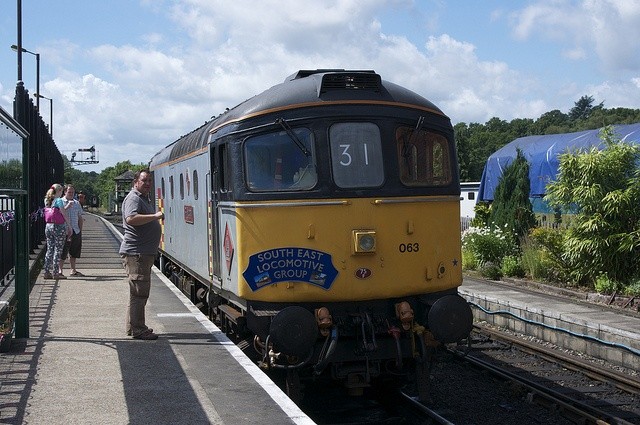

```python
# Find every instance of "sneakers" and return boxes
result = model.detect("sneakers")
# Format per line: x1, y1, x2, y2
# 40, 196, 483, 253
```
44, 274, 53, 279
53, 274, 66, 279
133, 332, 158, 339
127, 326, 153, 336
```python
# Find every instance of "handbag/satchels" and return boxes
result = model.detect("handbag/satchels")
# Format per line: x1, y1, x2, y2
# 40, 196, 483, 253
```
44, 206, 65, 225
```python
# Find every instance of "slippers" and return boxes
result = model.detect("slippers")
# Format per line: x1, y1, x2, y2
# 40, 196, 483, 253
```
70, 271, 85, 277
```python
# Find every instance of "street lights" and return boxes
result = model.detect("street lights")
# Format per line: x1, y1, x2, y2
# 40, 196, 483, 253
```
10, 44, 39, 111
33, 93, 53, 136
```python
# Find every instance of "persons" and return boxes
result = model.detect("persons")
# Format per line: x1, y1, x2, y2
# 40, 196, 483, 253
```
44, 183, 73, 280
78, 190, 85, 208
119, 170, 165, 342
58, 184, 86, 279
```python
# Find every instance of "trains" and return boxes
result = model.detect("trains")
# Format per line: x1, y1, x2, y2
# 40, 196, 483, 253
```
147, 69, 473, 398
